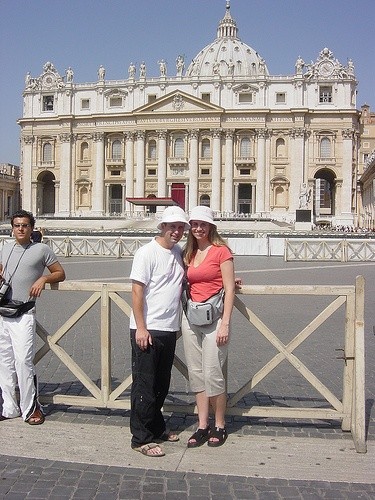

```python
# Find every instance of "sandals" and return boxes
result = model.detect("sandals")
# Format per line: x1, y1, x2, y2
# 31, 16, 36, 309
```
28, 408, 45, 425
0, 412, 22, 421
187, 425, 214, 448
208, 426, 228, 447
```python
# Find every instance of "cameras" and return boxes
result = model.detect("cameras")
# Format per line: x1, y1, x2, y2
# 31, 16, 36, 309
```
0, 283, 11, 301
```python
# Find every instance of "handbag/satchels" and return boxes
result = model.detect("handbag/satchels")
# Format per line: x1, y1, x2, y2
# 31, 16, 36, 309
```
181, 284, 225, 326
0, 300, 35, 318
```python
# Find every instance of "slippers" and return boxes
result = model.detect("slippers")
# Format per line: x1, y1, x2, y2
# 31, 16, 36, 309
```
132, 441, 165, 457
159, 431, 179, 441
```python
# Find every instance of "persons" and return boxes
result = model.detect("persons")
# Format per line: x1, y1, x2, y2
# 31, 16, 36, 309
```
140, 61, 146, 76
30, 226, 44, 243
212, 58, 220, 75
181, 206, 235, 448
191, 59, 200, 74
98, 64, 105, 79
25, 71, 41, 90
129, 206, 241, 457
0, 209, 66, 425
176, 55, 184, 72
55, 67, 74, 88
158, 59, 168, 76
335, 59, 354, 79
314, 224, 375, 232
298, 183, 311, 208
128, 62, 136, 77
225, 59, 234, 75
295, 56, 320, 80
259, 57, 267, 75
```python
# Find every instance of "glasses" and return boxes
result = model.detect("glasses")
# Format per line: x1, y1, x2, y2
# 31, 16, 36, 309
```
13, 224, 31, 227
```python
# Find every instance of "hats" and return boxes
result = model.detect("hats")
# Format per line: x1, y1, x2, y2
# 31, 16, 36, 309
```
157, 206, 191, 232
188, 206, 217, 230
